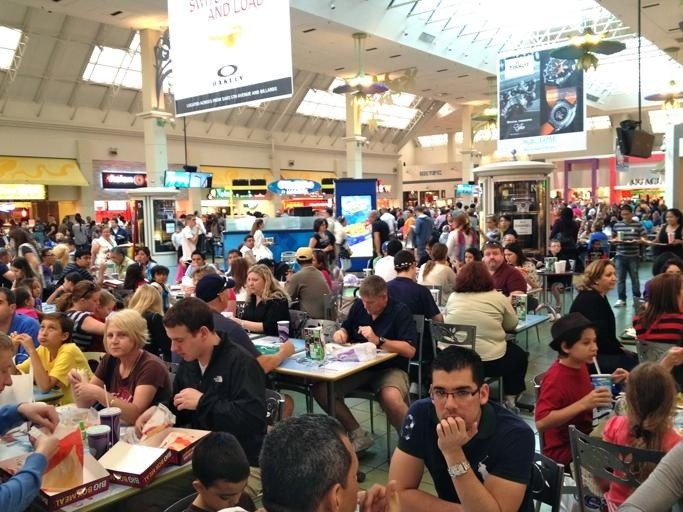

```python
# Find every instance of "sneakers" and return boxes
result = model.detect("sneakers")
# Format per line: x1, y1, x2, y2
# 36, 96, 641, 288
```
346, 431, 375, 452
633, 296, 642, 303
615, 300, 626, 307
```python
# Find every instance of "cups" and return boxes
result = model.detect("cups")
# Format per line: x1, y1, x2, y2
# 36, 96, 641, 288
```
554, 262, 562, 274
86, 425, 111, 460
429, 288, 440, 306
362, 268, 372, 278
99, 407, 122, 449
221, 311, 234, 320
667, 233, 676, 244
559, 259, 567, 272
589, 374, 614, 411
617, 231, 625, 241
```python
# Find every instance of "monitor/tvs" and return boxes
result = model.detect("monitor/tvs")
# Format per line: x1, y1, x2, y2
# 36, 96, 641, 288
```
614, 127, 655, 159
163, 170, 214, 189
163, 221, 177, 235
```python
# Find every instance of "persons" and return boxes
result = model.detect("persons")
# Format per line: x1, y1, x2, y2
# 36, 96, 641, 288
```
619, 441, 682, 511
0, 332, 61, 512
387, 345, 536, 511
258, 412, 402, 511
601, 345, 683, 512
312, 275, 419, 450
68, 307, 173, 427
134, 295, 270, 468
181, 431, 264, 512
2, 188, 683, 414
533, 311, 630, 468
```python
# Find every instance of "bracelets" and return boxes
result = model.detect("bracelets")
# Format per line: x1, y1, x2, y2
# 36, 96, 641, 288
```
448, 459, 472, 479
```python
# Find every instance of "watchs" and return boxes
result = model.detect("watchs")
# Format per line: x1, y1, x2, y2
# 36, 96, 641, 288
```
541, 98, 578, 135
543, 55, 579, 109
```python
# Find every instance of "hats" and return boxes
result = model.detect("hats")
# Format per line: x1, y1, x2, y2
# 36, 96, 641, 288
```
196, 274, 235, 302
549, 313, 592, 350
296, 246, 313, 261
653, 251, 683, 276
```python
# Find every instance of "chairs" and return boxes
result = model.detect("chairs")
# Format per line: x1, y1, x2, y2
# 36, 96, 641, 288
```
532, 296, 683, 508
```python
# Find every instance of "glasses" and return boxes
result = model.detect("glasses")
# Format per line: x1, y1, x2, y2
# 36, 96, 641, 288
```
80, 283, 96, 298
483, 240, 501, 247
429, 385, 479, 402
395, 261, 413, 270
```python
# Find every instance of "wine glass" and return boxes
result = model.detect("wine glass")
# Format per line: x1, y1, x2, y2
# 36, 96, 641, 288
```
276, 320, 290, 344
